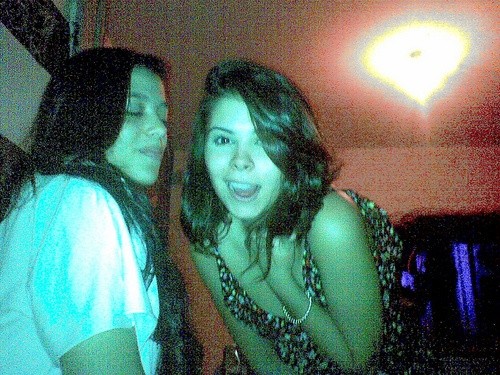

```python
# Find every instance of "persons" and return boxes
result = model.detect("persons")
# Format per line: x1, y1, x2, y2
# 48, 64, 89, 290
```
0, 47, 174, 375
179, 62, 424, 375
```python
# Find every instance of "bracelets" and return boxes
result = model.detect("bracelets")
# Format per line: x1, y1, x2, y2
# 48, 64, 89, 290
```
283, 296, 314, 325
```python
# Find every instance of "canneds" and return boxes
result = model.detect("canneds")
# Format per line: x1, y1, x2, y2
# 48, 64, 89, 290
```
222, 343, 242, 375
